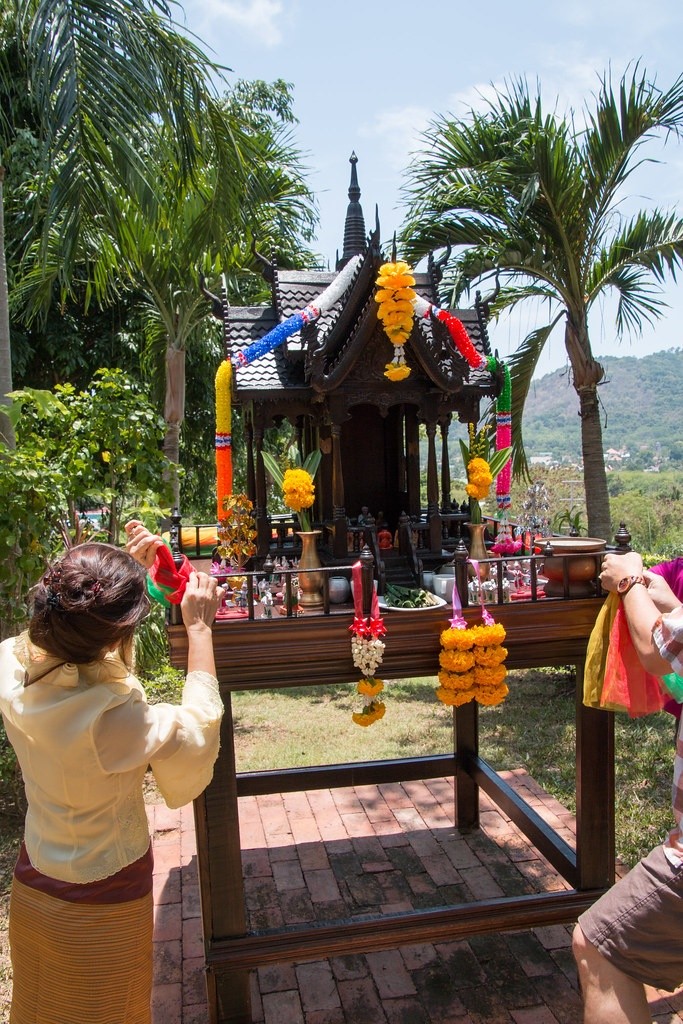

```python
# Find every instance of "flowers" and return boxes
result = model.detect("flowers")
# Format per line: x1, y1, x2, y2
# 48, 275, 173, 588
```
434, 623, 510, 707
350, 634, 387, 727
259, 444, 323, 533
458, 422, 514, 524
374, 262, 419, 383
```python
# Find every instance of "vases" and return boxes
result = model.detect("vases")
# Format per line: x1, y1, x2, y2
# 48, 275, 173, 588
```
294, 530, 325, 607
462, 522, 491, 585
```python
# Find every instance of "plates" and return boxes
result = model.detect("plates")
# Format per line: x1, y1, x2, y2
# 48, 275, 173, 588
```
377, 593, 447, 611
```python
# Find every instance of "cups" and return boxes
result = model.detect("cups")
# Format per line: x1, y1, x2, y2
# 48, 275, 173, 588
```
432, 574, 456, 602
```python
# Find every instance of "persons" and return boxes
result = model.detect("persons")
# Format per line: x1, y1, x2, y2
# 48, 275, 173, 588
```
376, 511, 388, 530
0, 519, 224, 1024
570, 550, 683, 1024
358, 506, 371, 526
